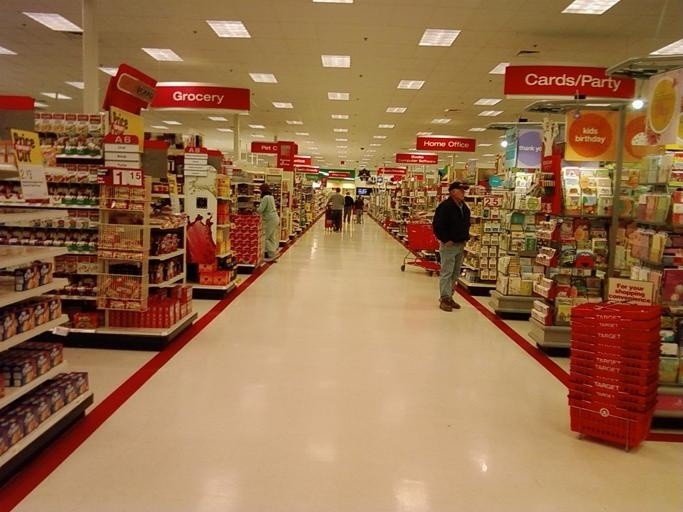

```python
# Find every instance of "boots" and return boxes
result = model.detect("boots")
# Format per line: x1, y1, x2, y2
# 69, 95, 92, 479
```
439, 297, 452, 311
450, 298, 460, 309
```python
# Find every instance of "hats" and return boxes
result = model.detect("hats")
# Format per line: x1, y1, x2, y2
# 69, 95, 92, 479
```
448, 182, 468, 191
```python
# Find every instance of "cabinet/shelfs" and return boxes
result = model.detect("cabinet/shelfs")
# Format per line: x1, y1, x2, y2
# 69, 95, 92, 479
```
2, 113, 330, 351
0, 164, 95, 471
365, 53, 683, 418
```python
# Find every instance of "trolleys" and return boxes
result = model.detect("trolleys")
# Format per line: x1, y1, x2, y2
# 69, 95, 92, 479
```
399, 216, 439, 277
352, 205, 364, 224
322, 204, 342, 231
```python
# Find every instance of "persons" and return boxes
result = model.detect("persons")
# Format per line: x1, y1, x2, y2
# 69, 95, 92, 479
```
327, 188, 345, 231
344, 192, 354, 224
256, 183, 280, 263
355, 195, 364, 224
432, 181, 470, 311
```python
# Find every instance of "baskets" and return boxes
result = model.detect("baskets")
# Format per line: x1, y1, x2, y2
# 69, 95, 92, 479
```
567, 303, 661, 448
